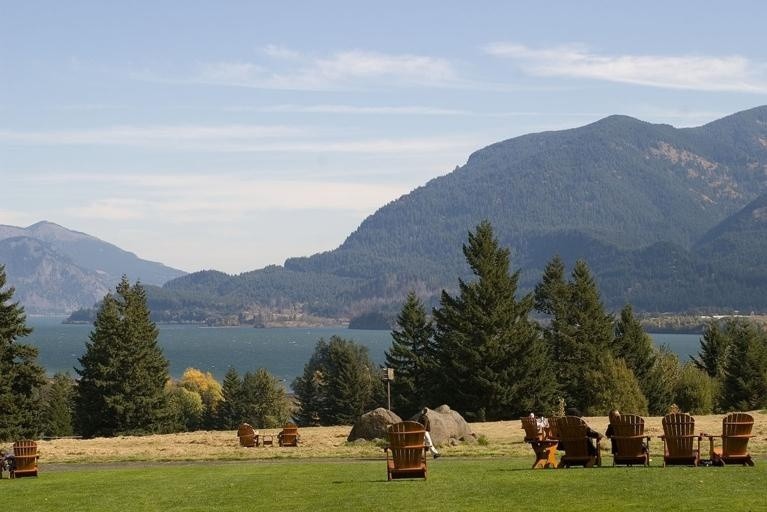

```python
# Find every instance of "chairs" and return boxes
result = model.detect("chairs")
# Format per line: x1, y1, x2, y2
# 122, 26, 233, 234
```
10, 440, 40, 478
384, 423, 432, 481
544, 417, 562, 440
277, 423, 300, 447
704, 413, 755, 466
609, 415, 651, 467
555, 416, 601, 469
237, 423, 260, 447
658, 413, 702, 467
521, 416, 543, 443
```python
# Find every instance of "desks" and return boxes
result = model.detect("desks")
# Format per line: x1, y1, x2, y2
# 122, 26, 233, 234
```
529, 440, 558, 469
263, 435, 273, 446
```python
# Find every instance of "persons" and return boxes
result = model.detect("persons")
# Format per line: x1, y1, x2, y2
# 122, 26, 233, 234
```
417, 408, 441, 460
563, 407, 601, 454
604, 408, 647, 455
